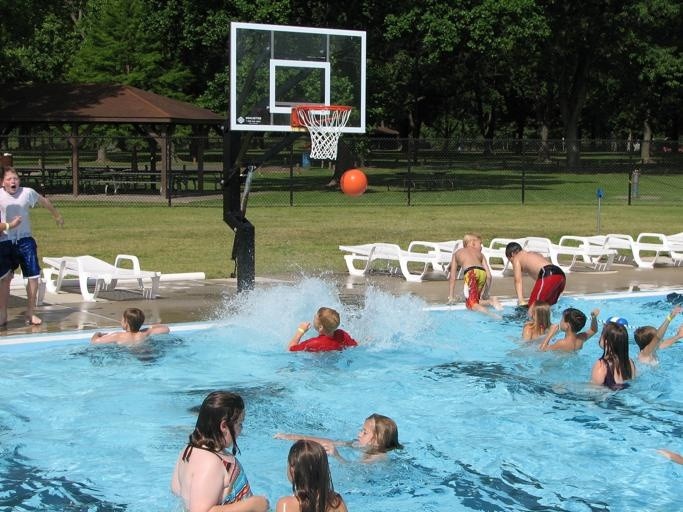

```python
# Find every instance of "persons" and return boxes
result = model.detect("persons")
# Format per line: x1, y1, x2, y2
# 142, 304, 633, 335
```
522, 301, 553, 342
1, 166, 64, 325
289, 307, 360, 352
92, 308, 171, 361
273, 414, 405, 464
506, 242, 567, 308
538, 308, 600, 351
171, 391, 269, 512
592, 323, 637, 391
277, 439, 347, 512
656, 449, 682, 465
447, 232, 504, 320
634, 303, 683, 366
1, 164, 23, 325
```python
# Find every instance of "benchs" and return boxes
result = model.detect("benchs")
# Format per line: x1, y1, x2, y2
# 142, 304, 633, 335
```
385, 176, 456, 192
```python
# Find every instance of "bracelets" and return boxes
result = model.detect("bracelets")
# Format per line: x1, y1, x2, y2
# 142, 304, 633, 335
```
54, 215, 63, 220
668, 316, 672, 322
298, 328, 305, 333
6, 223, 10, 229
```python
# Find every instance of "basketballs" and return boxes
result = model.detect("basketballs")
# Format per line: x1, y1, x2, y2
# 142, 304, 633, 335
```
340, 169, 367, 195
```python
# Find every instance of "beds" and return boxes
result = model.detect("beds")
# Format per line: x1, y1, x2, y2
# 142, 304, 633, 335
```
406, 240, 509, 278
337, 243, 452, 283
557, 232, 682, 270
40, 254, 161, 302
489, 237, 616, 273
